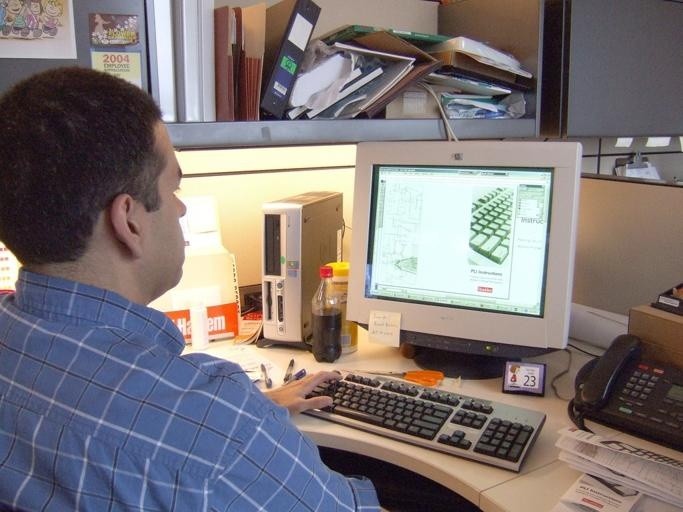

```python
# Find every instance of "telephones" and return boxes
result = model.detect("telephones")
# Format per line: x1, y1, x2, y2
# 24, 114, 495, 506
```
574, 334, 683, 452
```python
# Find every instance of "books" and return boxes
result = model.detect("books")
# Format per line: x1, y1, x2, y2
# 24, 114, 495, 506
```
553, 425, 682, 508
547, 470, 646, 512
216, 2, 535, 120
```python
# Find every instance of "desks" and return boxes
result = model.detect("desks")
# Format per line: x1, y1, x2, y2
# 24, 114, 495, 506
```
184, 334, 683, 512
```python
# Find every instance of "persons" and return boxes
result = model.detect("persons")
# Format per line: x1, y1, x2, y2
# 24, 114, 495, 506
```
0, 62, 394, 512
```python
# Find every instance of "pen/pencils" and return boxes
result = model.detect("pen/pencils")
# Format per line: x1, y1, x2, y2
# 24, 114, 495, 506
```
261, 364, 272, 389
282, 358, 306, 386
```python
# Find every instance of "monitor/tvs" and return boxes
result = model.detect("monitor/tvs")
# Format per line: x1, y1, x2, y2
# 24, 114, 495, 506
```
346, 140, 583, 379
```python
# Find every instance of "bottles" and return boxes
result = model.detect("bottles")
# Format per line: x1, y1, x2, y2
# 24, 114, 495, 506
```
311, 262, 358, 364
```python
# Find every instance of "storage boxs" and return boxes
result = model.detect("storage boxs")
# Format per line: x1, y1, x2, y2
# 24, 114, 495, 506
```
627, 303, 683, 370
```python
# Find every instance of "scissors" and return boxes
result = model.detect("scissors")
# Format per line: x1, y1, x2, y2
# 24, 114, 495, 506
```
357, 370, 444, 387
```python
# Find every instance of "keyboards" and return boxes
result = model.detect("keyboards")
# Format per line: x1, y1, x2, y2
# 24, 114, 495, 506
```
301, 370, 546, 473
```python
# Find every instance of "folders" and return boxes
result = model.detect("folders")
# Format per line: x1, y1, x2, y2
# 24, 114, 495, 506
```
260, 0, 322, 120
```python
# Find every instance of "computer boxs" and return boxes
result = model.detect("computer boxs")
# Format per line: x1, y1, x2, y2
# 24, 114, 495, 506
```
256, 192, 343, 352
